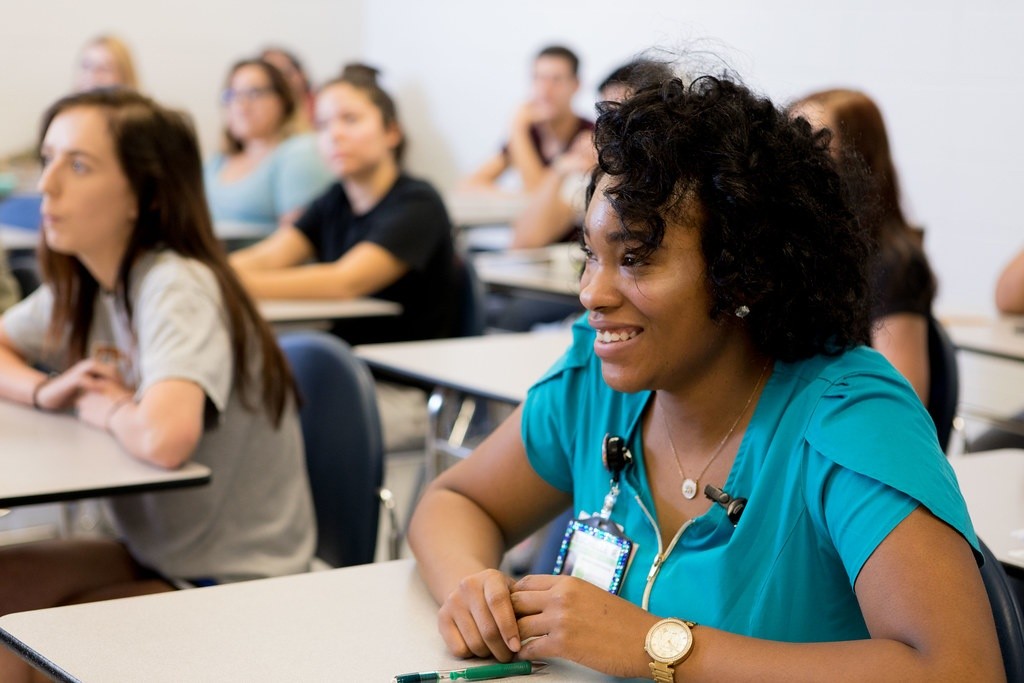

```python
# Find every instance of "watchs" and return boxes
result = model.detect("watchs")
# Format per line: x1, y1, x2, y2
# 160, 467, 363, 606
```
643, 616, 698, 683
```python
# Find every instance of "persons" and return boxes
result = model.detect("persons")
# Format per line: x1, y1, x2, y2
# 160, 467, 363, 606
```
221, 79, 479, 450
0, 87, 314, 683
408, 76, 1005, 683
201, 49, 337, 251
965, 251, 1024, 456
776, 89, 936, 407
449, 62, 674, 438
472, 46, 594, 250
0, 36, 138, 301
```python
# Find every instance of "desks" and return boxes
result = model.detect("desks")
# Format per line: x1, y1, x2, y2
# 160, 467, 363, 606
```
0, 217, 1024, 683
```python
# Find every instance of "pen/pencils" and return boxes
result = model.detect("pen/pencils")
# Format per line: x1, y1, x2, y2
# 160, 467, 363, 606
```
392, 660, 549, 683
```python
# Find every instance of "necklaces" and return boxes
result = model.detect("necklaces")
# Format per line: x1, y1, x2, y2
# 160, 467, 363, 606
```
659, 369, 766, 500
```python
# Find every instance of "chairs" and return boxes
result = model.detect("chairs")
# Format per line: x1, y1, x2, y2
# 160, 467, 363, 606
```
275, 329, 403, 573
402, 258, 494, 536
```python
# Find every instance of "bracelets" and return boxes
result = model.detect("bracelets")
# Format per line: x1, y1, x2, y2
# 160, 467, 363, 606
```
32, 376, 53, 411
